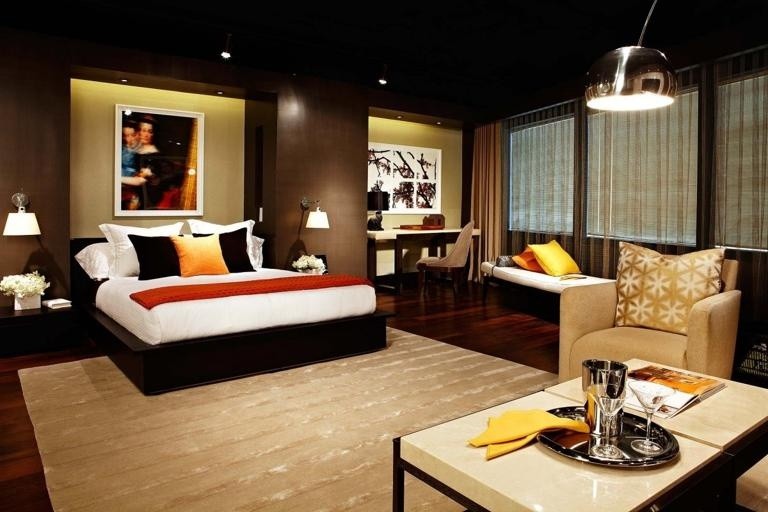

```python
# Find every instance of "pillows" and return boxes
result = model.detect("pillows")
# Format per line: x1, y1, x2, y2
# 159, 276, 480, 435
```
525, 239, 582, 275
495, 255, 515, 268
75, 220, 256, 283
510, 244, 547, 274
614, 240, 724, 337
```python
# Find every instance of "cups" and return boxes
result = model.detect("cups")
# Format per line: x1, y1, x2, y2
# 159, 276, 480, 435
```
581, 358, 628, 436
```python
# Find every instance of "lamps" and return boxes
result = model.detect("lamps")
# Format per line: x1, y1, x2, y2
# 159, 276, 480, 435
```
367, 191, 390, 230
573, 0, 681, 116
2, 193, 43, 237
299, 196, 330, 231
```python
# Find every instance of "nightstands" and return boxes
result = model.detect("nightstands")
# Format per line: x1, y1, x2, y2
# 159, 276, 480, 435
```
0, 303, 70, 357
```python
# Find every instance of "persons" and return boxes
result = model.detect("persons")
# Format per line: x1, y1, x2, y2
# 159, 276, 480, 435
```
119, 112, 168, 209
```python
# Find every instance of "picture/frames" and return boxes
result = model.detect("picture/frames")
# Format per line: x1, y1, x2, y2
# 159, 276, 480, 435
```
368, 142, 442, 216
111, 103, 203, 219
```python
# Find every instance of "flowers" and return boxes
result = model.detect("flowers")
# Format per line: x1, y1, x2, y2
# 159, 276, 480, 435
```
289, 253, 328, 276
0, 269, 48, 298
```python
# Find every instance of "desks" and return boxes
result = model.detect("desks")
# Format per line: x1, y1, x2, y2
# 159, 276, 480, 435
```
367, 225, 480, 300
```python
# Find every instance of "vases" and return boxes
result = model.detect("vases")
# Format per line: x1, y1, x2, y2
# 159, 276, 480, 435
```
12, 294, 43, 311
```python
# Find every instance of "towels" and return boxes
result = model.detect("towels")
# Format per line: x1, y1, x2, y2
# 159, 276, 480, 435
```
465, 408, 589, 462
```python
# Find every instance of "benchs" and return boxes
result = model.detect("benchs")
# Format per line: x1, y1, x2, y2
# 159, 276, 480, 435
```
480, 255, 617, 318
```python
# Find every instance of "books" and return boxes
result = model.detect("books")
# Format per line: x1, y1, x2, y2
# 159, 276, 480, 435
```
41, 297, 72, 310
623, 363, 728, 421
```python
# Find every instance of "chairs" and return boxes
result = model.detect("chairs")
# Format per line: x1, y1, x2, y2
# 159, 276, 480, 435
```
560, 256, 740, 379
414, 216, 474, 300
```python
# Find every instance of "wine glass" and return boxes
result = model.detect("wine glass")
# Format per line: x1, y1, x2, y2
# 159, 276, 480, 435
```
628, 379, 677, 455
586, 383, 630, 461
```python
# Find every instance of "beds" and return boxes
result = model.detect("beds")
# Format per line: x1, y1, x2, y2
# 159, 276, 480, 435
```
69, 221, 389, 392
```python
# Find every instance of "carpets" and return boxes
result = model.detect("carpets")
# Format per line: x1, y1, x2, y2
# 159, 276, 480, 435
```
17, 327, 560, 511
